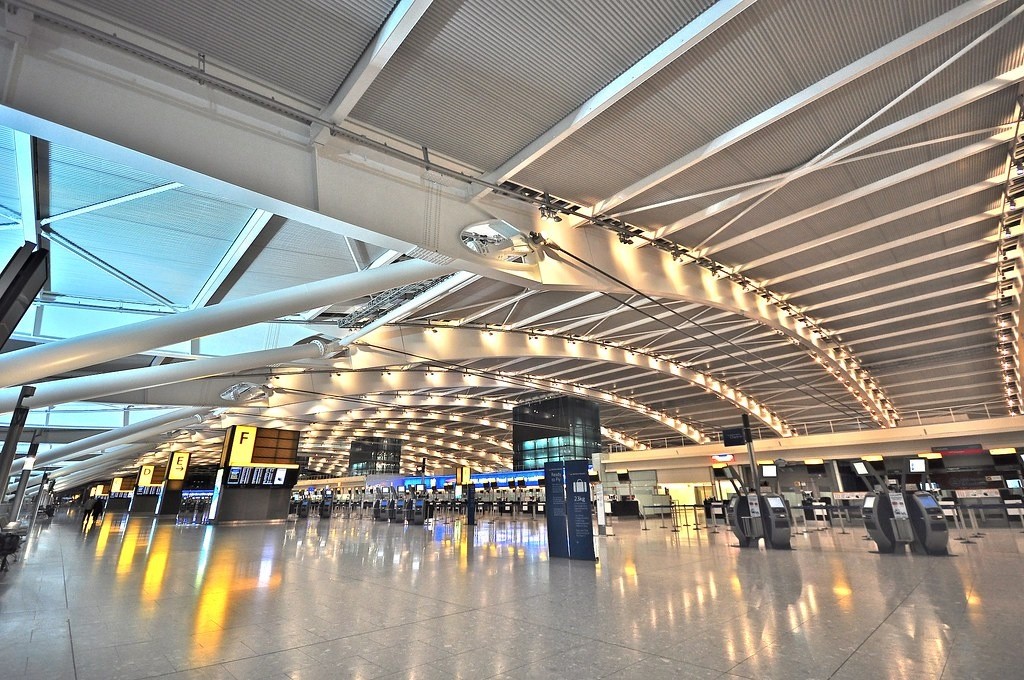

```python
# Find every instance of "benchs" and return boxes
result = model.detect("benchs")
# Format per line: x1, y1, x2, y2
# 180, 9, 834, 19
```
0, 532, 21, 573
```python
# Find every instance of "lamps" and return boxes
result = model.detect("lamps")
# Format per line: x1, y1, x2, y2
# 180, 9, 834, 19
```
440, 93, 1021, 449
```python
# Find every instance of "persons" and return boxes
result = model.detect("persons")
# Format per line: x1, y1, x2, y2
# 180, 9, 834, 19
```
91, 498, 103, 521
82, 496, 95, 521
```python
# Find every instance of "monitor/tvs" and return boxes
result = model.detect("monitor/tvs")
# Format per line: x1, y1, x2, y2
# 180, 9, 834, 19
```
917, 494, 939, 507
302, 501, 307, 505
397, 500, 404, 508
767, 497, 784, 507
325, 501, 330, 506
729, 497, 737, 507
864, 497, 876, 507
853, 462, 868, 474
415, 500, 422, 508
1006, 479, 1022, 488
398, 486, 404, 492
325, 491, 332, 498
417, 485, 424, 491
382, 487, 389, 492
762, 465, 776, 477
905, 458, 927, 474
381, 500, 387, 507
